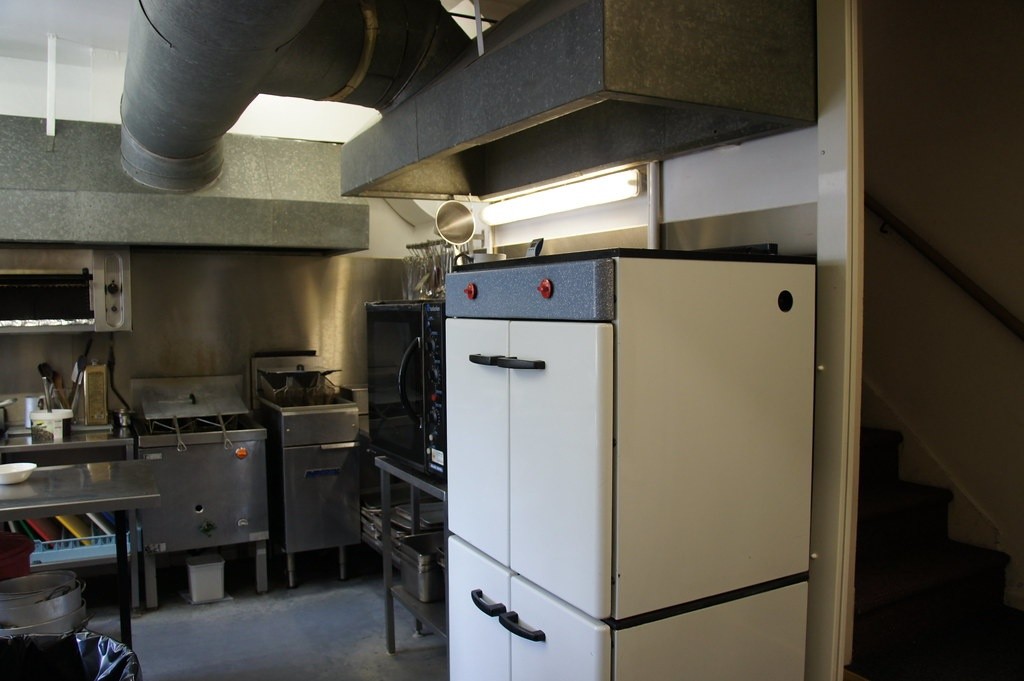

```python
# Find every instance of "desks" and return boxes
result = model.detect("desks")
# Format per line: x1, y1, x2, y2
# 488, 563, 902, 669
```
0, 460, 162, 650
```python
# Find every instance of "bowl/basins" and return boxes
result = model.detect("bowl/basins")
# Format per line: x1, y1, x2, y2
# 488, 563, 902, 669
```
0, 463, 37, 484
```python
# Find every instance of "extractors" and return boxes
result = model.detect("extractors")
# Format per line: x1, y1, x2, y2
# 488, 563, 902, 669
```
0, 245, 133, 335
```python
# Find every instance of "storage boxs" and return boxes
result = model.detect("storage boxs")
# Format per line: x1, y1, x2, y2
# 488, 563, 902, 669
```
1, 533, 36, 580
391, 531, 450, 603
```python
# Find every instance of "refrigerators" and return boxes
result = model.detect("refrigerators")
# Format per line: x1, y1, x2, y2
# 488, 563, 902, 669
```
446, 257, 817, 681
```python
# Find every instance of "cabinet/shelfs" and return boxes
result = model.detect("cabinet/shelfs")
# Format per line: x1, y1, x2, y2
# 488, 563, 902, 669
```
0, 425, 140, 609
373, 456, 448, 655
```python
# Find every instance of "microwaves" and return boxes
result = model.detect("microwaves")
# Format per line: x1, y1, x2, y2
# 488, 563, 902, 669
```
365, 299, 446, 478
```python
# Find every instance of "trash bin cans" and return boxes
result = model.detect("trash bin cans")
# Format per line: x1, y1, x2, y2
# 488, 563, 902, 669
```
0, 631, 144, 681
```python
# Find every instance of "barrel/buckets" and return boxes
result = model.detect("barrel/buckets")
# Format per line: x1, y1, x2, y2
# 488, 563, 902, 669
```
0, 570, 96, 637
